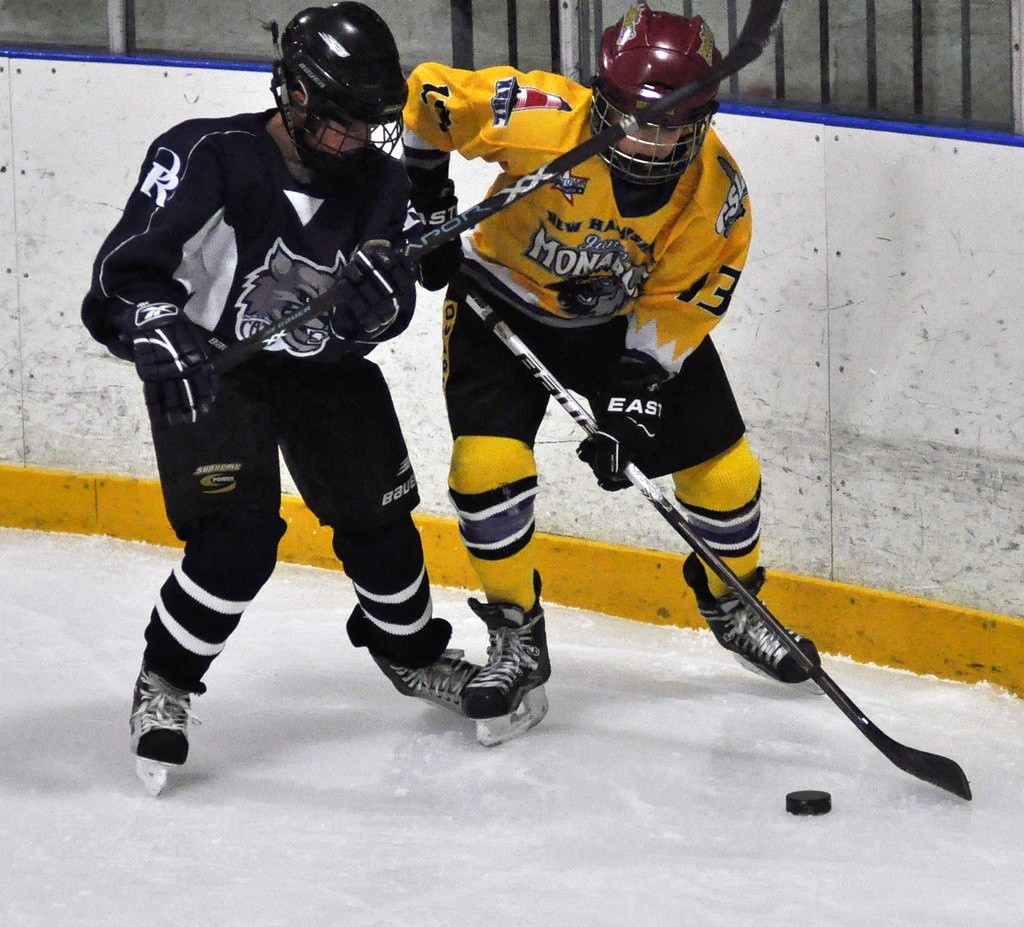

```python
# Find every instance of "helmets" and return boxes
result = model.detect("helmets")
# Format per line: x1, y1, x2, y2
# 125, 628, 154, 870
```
588, 6, 725, 185
270, 0, 409, 174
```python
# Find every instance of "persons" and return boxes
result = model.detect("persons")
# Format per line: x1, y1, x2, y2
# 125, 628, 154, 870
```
81, 2, 484, 794
396, 1, 827, 748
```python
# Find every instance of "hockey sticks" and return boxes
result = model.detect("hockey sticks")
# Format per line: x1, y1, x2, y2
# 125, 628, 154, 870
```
467, 290, 977, 807
200, 1, 783, 382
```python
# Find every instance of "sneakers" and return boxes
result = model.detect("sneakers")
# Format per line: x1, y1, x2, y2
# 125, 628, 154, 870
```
463, 569, 551, 747
346, 606, 484, 718
683, 552, 825, 695
129, 660, 208, 797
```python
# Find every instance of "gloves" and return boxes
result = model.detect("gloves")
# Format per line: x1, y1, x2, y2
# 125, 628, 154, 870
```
330, 238, 409, 342
405, 179, 465, 291
576, 348, 679, 482
128, 304, 218, 427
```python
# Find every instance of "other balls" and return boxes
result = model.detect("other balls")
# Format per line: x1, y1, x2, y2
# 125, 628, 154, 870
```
787, 789, 834, 812
